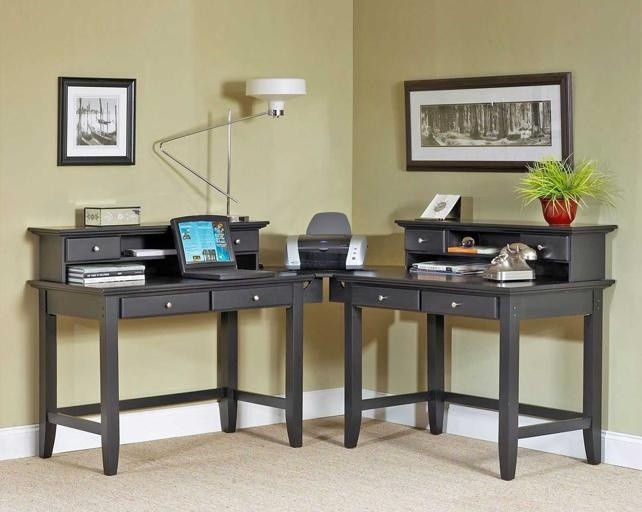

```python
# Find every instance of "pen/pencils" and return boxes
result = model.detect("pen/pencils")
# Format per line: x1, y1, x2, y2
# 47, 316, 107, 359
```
415, 218, 442, 220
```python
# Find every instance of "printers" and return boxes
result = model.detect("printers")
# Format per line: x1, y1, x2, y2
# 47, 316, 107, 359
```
284, 212, 368, 270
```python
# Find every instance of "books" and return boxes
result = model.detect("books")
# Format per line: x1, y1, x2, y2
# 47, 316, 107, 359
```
447, 246, 500, 255
409, 266, 486, 276
123, 247, 177, 257
67, 262, 147, 272
67, 274, 147, 284
413, 259, 491, 271
66, 270, 146, 279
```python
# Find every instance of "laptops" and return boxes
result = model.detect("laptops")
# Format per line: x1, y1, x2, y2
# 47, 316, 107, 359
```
170, 215, 276, 281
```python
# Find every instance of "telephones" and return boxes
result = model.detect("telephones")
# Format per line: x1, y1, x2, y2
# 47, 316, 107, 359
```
482, 243, 538, 282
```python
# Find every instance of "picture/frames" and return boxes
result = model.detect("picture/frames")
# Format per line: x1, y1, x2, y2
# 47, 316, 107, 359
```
56, 76, 138, 167
404, 71, 573, 173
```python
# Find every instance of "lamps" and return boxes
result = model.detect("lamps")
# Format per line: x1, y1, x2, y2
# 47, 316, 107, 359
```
152, 75, 309, 222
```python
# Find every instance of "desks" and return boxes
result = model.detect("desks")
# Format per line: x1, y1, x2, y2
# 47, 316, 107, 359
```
331, 218, 618, 480
18, 226, 319, 478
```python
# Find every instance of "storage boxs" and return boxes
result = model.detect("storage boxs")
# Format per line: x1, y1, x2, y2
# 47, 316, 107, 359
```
83, 206, 141, 228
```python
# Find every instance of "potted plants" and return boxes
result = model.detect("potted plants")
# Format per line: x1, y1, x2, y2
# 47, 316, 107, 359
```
513, 155, 619, 227
